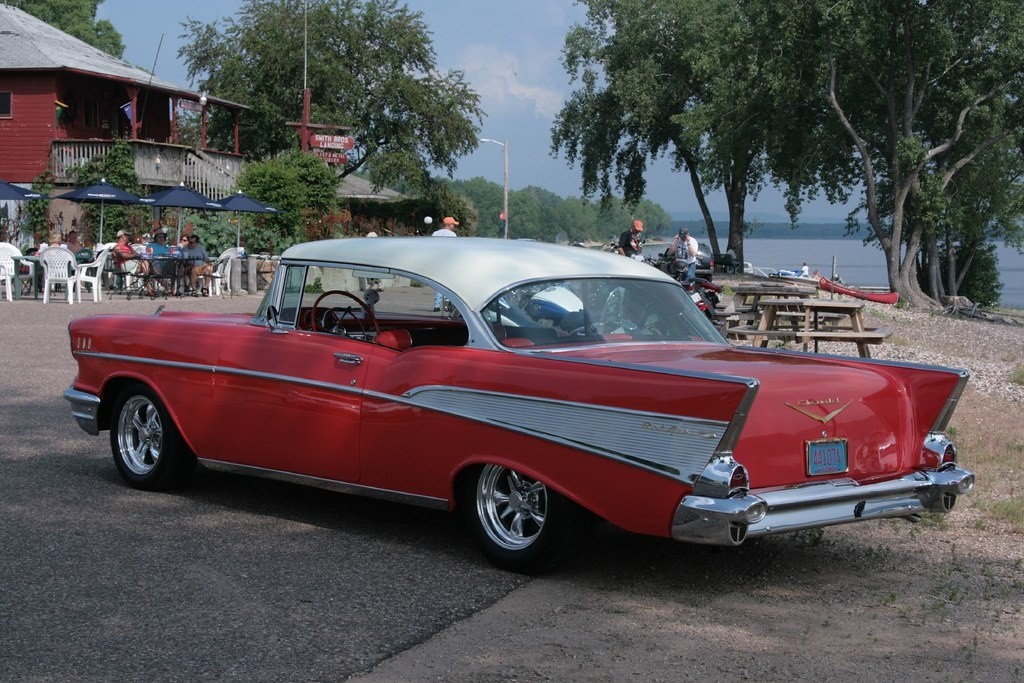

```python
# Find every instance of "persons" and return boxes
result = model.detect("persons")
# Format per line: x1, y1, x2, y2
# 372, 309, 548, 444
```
67, 230, 92, 254
364, 223, 385, 292
111, 229, 247, 297
430, 217, 459, 312
616, 220, 643, 257
58, 210, 65, 240
799, 262, 821, 280
669, 227, 698, 279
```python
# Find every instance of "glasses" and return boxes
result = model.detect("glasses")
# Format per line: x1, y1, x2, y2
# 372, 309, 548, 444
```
189, 241, 194, 244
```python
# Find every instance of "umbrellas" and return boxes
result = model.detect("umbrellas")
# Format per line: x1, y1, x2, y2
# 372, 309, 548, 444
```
137, 181, 224, 246
52, 178, 142, 243
216, 189, 284, 252
0, 180, 54, 200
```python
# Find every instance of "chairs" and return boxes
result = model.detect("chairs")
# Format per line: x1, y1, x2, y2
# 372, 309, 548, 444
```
374, 325, 469, 351
0, 242, 244, 304
491, 324, 559, 341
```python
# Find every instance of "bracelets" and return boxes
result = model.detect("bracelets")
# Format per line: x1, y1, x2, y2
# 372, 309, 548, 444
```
687, 244, 690, 246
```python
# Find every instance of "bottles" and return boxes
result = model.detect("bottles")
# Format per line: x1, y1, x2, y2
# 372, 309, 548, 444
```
170, 247, 173, 256
148, 246, 153, 255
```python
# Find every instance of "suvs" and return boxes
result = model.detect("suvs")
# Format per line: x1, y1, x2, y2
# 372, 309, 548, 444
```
638, 242, 716, 283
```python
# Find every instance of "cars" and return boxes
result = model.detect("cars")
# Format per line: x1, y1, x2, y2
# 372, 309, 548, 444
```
59, 233, 978, 575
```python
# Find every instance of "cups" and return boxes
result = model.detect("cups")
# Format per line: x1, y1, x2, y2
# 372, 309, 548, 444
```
142, 246, 146, 253
183, 252, 189, 258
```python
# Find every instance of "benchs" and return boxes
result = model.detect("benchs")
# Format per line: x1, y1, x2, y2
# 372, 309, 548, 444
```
500, 335, 705, 349
714, 300, 895, 337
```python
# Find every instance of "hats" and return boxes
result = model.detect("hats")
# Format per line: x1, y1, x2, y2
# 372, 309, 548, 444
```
153, 229, 167, 238
633, 220, 644, 231
117, 229, 133, 239
444, 217, 459, 224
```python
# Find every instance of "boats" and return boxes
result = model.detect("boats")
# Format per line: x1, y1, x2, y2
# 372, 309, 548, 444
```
818, 276, 900, 304
769, 269, 819, 288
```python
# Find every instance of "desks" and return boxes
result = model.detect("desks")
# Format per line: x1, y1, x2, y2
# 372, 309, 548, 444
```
722, 283, 872, 360
133, 256, 204, 299
10, 257, 91, 299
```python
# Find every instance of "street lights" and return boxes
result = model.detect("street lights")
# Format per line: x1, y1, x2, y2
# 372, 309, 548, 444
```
478, 138, 511, 240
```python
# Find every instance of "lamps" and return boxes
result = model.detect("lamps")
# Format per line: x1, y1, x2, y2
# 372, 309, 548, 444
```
199, 91, 207, 105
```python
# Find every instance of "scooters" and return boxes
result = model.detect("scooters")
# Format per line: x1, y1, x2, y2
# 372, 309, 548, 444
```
440, 241, 620, 320
643, 246, 725, 322
